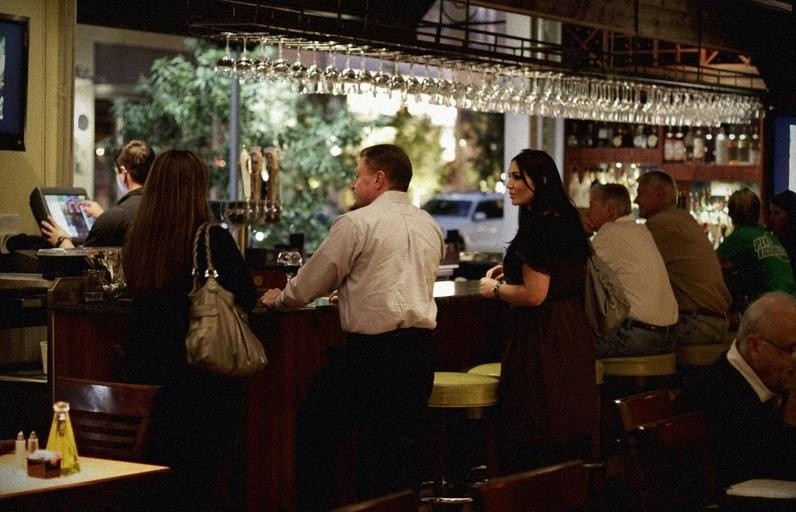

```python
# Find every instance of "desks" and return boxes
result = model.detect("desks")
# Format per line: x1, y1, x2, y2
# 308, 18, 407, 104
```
0, 445, 168, 510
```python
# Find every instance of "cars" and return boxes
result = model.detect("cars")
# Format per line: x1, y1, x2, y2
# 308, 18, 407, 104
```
414, 191, 507, 254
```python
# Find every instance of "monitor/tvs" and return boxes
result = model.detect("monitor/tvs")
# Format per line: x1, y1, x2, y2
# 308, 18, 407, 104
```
29, 187, 98, 245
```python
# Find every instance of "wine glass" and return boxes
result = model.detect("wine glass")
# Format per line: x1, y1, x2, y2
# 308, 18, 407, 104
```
275, 251, 303, 281
214, 28, 761, 130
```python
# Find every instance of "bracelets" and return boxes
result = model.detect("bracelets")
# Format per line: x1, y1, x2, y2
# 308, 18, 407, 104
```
492, 280, 504, 299
54, 235, 70, 247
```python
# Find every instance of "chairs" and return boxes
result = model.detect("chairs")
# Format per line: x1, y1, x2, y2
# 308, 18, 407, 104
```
55, 375, 162, 461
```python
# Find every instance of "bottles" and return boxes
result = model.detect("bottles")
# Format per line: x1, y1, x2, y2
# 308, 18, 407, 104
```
45, 400, 81, 477
567, 119, 760, 167
567, 164, 643, 207
14, 431, 38, 469
676, 186, 733, 249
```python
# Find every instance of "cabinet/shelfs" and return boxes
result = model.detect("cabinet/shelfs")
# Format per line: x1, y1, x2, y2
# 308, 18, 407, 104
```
567, 73, 659, 213
661, 88, 773, 250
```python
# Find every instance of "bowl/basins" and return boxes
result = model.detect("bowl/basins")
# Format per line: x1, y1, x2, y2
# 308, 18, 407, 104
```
36, 246, 113, 282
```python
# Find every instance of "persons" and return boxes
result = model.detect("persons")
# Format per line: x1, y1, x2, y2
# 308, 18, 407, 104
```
668, 290, 796, 511
38, 139, 156, 249
633, 171, 734, 343
259, 144, 446, 511
120, 148, 259, 512
573, 183, 680, 360
478, 148, 638, 470
768, 191, 796, 277
713, 188, 796, 328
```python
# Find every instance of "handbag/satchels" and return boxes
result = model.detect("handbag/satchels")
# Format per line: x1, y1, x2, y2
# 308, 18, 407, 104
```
184, 274, 269, 379
583, 253, 632, 334
